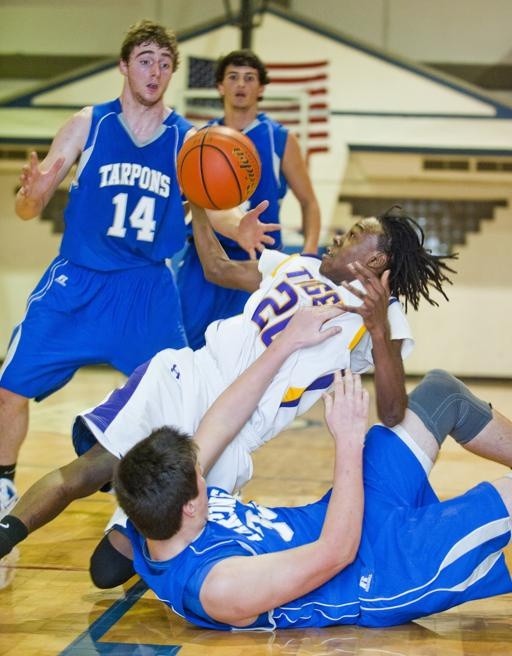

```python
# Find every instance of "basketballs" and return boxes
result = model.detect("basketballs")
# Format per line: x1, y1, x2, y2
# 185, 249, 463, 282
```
176, 127, 261, 210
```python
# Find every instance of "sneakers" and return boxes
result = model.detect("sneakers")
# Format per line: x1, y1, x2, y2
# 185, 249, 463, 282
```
0, 477, 19, 518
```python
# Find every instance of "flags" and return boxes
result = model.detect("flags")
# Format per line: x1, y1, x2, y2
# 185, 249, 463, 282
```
185, 52, 330, 152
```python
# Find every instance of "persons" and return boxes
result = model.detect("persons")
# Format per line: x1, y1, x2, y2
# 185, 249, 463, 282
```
169, 47, 323, 349
0, 189, 465, 592
109, 299, 512, 632
0, 20, 283, 525
